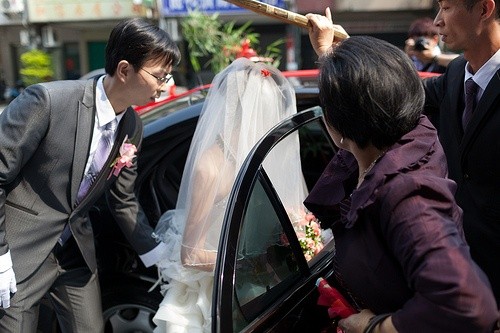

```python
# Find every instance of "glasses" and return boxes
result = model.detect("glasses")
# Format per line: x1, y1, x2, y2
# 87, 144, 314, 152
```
129, 61, 173, 86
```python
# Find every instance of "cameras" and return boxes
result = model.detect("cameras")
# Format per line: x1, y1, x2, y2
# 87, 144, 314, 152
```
413, 37, 430, 52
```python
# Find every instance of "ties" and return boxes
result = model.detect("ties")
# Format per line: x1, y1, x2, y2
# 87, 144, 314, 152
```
462, 79, 479, 133
57, 117, 119, 247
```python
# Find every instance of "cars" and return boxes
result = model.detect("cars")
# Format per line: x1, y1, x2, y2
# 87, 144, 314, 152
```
42, 76, 337, 333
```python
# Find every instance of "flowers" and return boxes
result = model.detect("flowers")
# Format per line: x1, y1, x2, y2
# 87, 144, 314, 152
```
106, 134, 140, 181
279, 208, 325, 261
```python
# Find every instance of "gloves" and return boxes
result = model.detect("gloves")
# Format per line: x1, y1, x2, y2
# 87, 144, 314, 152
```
157, 260, 171, 282
0, 248, 18, 309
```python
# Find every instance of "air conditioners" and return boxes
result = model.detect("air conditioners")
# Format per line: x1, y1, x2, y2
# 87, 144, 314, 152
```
41, 24, 65, 48
0, 0, 25, 15
18, 28, 31, 48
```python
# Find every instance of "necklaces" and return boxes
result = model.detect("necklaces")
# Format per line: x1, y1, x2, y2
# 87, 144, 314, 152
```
358, 151, 385, 179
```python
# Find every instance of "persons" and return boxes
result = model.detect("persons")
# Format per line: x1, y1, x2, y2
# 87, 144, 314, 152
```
144, 58, 309, 333
333, 0, 500, 315
299, 6, 500, 333
404, 18, 464, 74
0, 16, 172, 333
